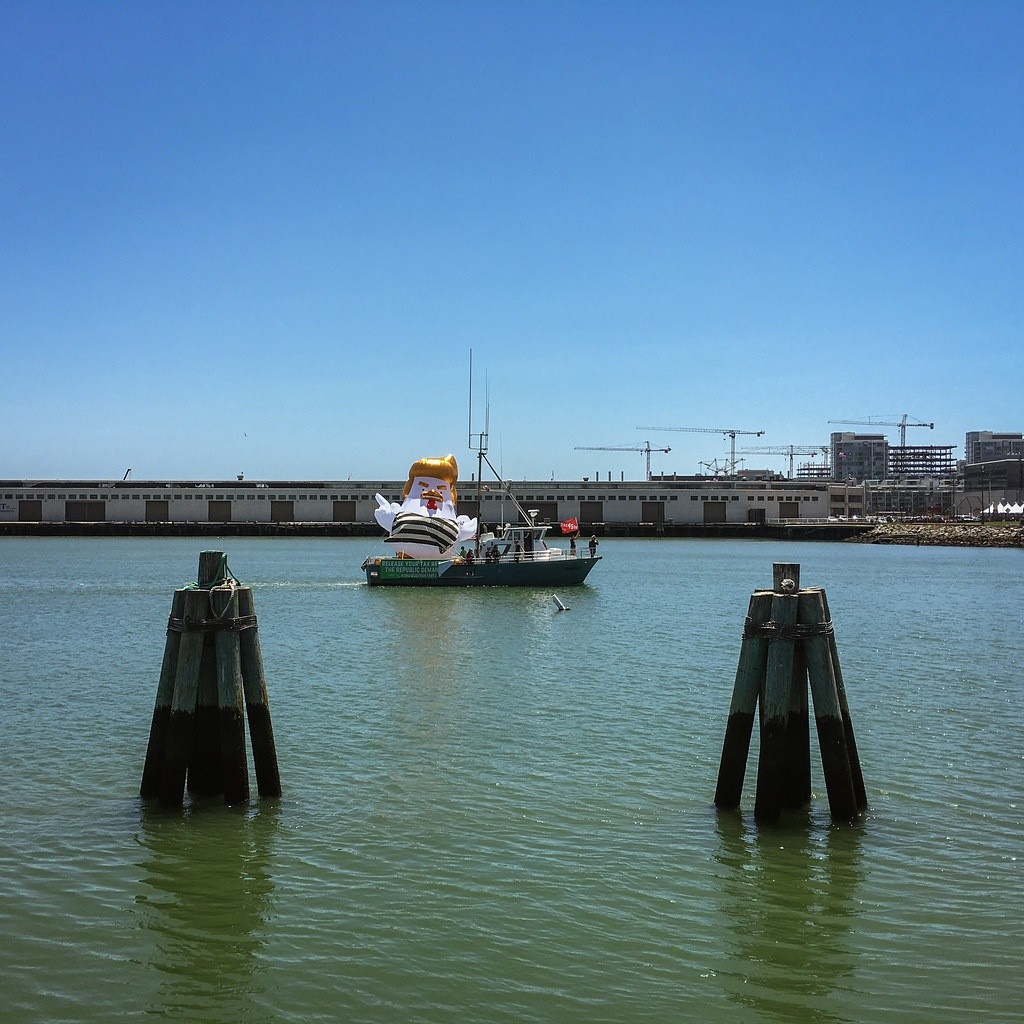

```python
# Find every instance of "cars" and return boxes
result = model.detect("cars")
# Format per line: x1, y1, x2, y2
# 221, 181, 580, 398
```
826, 512, 975, 523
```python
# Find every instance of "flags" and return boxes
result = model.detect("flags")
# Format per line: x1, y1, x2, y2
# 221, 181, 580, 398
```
560, 516, 580, 533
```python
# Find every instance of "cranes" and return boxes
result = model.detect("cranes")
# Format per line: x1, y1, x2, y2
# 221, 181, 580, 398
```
573, 440, 672, 481
740, 444, 832, 477
636, 425, 766, 481
724, 444, 818, 481
828, 412, 935, 471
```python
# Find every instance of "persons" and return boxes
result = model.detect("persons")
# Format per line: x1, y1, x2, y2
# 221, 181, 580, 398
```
589, 535, 599, 557
514, 540, 525, 562
485, 544, 501, 564
524, 531, 532, 551
460, 546, 474, 564
569, 530, 580, 556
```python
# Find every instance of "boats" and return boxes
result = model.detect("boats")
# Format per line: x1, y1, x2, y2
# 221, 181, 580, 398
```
360, 347, 604, 589
